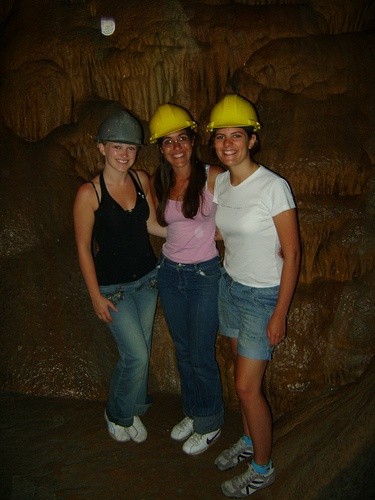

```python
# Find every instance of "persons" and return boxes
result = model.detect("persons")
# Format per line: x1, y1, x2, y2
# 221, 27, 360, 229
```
72, 112, 166, 442
147, 102, 224, 455
207, 94, 301, 497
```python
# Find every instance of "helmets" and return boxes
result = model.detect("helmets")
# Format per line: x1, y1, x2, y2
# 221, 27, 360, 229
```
205, 94, 261, 132
149, 104, 197, 144
95, 110, 146, 147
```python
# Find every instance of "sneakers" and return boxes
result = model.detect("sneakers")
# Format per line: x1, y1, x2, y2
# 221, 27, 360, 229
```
125, 415, 148, 443
220, 462, 275, 497
170, 416, 194, 441
214, 437, 254, 472
103, 408, 130, 442
182, 428, 222, 456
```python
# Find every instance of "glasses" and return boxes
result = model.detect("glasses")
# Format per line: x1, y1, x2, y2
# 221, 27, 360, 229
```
161, 135, 192, 146
